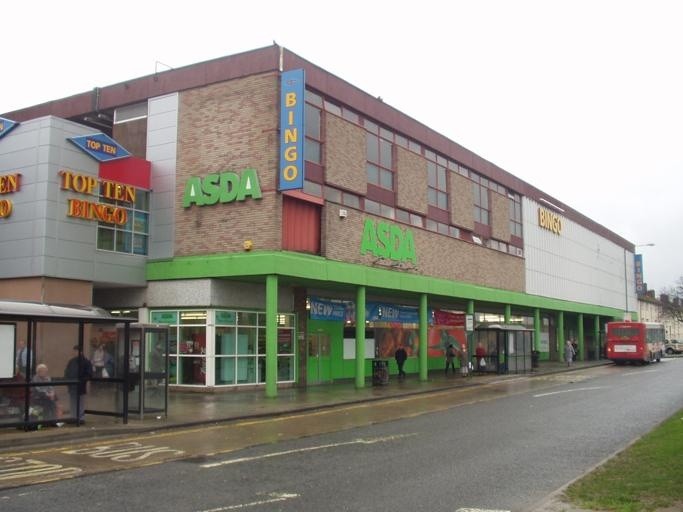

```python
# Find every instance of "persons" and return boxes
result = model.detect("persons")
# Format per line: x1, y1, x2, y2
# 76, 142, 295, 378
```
497, 349, 506, 374
460, 343, 468, 376
28, 364, 57, 420
15, 338, 32, 381
476, 342, 486, 369
564, 340, 575, 367
93, 343, 105, 379
148, 344, 162, 384
445, 344, 455, 373
395, 344, 407, 376
64, 346, 91, 424
429, 330, 461, 355
571, 338, 578, 361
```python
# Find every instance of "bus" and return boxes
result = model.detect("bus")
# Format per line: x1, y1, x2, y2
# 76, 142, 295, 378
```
606, 321, 666, 365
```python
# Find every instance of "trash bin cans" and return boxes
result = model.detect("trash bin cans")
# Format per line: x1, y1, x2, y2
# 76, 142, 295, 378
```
372, 359, 390, 386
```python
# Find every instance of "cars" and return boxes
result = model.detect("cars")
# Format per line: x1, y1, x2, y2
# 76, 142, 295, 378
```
665, 339, 683, 355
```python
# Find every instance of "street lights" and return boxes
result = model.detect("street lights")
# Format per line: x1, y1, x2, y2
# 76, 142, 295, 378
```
623, 242, 656, 320
